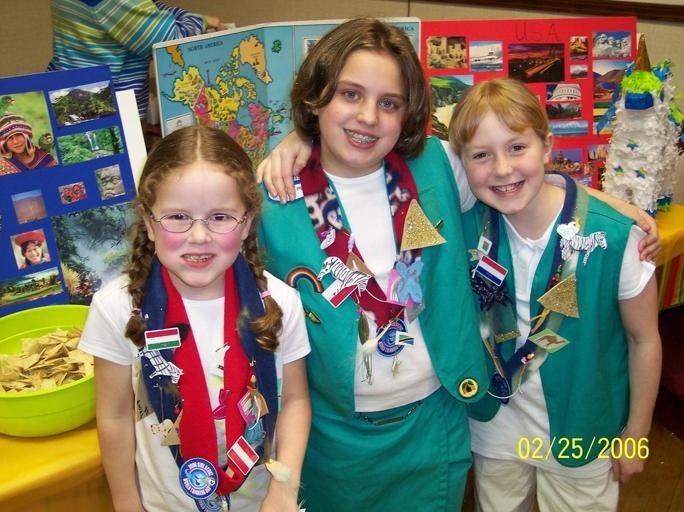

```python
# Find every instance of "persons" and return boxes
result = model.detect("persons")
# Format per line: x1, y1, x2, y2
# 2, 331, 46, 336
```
100, 164, 125, 198
0, 114, 57, 176
15, 230, 51, 269
45, 0, 227, 128
76, 124, 313, 511
62, 183, 87, 206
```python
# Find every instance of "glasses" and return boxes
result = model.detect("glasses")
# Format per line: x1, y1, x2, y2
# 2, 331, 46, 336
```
143, 204, 246, 233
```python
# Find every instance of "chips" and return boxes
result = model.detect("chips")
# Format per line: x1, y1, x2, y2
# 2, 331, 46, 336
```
0, 328, 95, 393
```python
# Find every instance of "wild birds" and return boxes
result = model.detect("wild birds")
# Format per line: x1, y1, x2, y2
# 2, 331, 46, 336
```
37, 132, 57, 154
1, 93, 17, 117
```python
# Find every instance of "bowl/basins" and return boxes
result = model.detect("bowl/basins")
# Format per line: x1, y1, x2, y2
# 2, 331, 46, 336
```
0, 304, 96, 438
